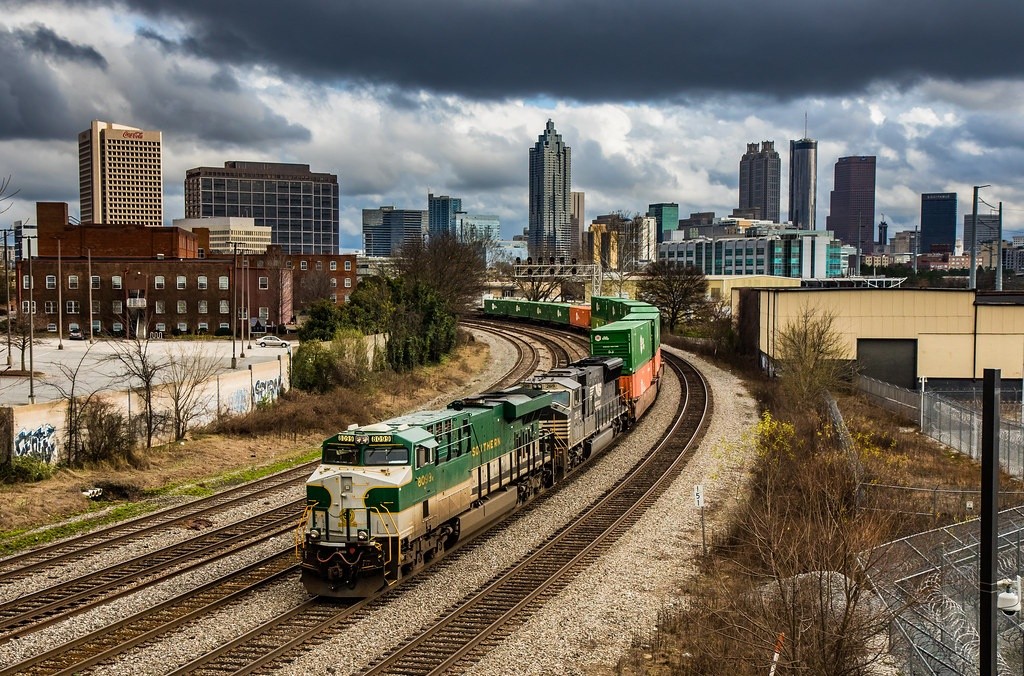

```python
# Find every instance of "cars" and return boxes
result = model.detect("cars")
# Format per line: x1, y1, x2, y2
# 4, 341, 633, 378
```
256, 336, 291, 348
69, 329, 86, 339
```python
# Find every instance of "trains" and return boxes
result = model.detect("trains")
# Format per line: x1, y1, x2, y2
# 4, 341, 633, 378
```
295, 295, 666, 594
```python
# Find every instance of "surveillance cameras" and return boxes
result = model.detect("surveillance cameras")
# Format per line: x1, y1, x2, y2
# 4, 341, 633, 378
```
997, 593, 1020, 616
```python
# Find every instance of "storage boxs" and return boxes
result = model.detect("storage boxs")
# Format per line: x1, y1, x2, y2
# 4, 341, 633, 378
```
483, 294, 661, 400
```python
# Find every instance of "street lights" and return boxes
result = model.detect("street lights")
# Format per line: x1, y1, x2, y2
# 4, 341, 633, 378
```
0, 228, 16, 365
17, 235, 40, 405
83, 246, 98, 344
979, 578, 1021, 676
226, 241, 251, 368
51, 236, 67, 350
454, 211, 467, 243
